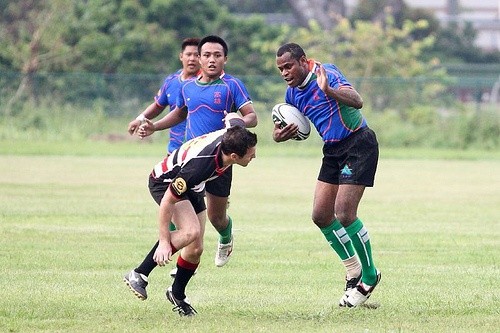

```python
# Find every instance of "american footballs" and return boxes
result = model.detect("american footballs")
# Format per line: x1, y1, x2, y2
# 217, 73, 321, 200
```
271, 102, 311, 142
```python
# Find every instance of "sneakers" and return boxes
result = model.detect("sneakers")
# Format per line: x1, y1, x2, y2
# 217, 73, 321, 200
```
215, 234, 233, 267
338, 268, 381, 308
166, 286, 199, 318
123, 268, 149, 301
170, 268, 197, 277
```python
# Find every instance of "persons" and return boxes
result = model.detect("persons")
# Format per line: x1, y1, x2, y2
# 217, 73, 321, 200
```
122, 35, 258, 318
273, 43, 383, 307
